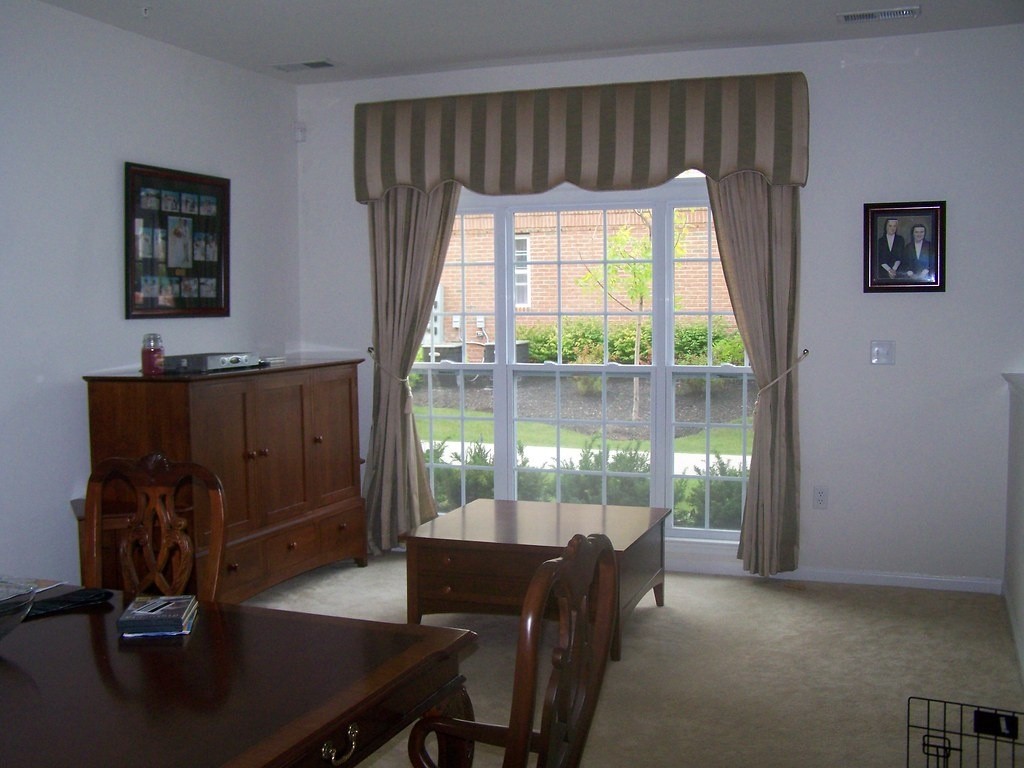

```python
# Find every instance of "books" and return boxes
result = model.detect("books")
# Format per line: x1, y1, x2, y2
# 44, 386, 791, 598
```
120, 593, 199, 638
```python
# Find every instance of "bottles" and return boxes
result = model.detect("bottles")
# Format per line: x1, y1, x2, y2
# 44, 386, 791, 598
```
141, 334, 164, 376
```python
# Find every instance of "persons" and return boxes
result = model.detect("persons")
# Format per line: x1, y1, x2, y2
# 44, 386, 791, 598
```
904, 223, 931, 279
141, 190, 218, 297
877, 218, 906, 278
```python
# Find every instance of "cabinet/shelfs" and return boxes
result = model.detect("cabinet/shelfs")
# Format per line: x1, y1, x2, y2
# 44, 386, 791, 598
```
81, 356, 369, 609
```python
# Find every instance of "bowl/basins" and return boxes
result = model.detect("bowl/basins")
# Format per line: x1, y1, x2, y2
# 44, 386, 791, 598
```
0, 580, 39, 639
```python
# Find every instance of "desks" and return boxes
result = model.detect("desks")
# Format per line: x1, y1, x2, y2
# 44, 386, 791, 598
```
398, 498, 672, 662
0, 577, 480, 768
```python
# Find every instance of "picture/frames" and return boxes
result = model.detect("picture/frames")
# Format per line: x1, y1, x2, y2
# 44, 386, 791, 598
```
863, 201, 945, 293
123, 161, 230, 319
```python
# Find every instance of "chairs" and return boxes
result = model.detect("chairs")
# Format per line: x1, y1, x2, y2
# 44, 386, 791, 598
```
80, 446, 225, 602
407, 532, 619, 768
